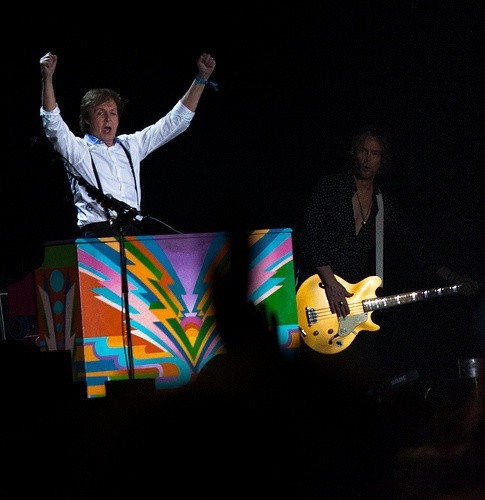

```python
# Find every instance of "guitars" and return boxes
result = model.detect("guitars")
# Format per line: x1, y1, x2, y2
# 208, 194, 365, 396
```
297, 270, 485, 355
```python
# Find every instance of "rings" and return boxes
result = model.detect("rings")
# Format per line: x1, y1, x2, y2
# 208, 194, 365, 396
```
338, 300, 344, 305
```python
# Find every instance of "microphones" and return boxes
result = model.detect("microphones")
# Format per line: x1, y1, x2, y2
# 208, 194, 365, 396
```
106, 194, 136, 212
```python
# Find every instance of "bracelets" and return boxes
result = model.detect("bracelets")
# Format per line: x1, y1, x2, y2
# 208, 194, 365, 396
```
356, 192, 374, 225
446, 270, 459, 284
195, 74, 219, 91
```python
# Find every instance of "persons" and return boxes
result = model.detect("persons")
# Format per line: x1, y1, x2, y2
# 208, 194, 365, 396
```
40, 52, 216, 238
301, 128, 481, 318
